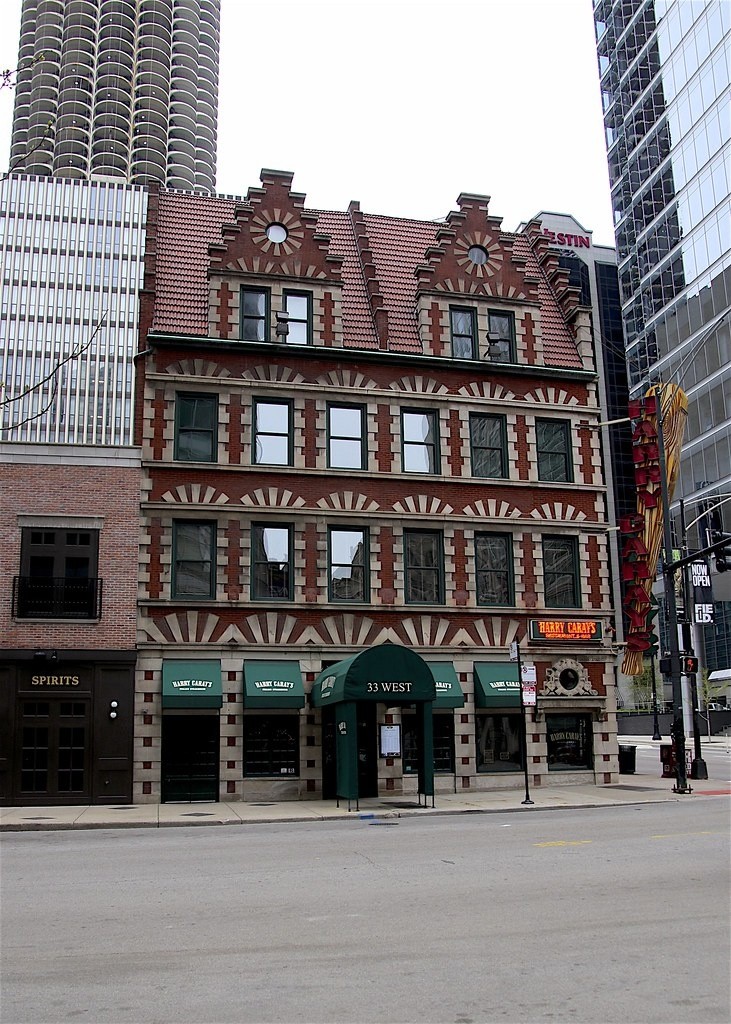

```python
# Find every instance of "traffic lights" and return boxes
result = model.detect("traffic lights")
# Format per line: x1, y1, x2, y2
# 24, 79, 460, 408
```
679, 656, 699, 676
712, 529, 731, 574
659, 658, 671, 673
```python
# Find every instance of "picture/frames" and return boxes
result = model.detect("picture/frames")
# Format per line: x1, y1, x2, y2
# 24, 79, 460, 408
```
499, 751, 510, 761
483, 749, 495, 765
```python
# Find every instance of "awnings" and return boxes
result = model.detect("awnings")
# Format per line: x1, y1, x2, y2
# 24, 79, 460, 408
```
473, 661, 522, 708
405, 662, 465, 709
243, 661, 305, 709
162, 660, 222, 708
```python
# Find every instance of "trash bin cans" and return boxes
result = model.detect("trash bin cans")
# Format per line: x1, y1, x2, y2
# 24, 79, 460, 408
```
619, 745, 637, 774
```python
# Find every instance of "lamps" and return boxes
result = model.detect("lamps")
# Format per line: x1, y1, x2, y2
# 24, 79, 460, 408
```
33, 652, 46, 656
483, 332, 502, 359
108, 700, 118, 719
50, 650, 57, 660
274, 311, 289, 338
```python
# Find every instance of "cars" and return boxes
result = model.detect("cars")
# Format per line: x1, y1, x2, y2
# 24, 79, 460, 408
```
708, 703, 719, 711
